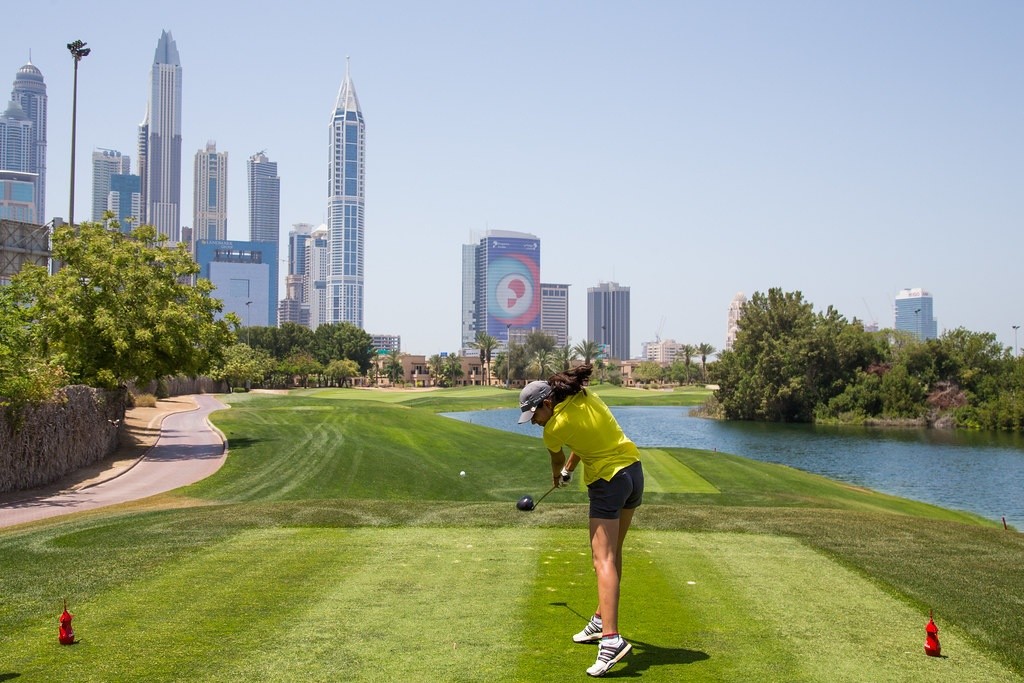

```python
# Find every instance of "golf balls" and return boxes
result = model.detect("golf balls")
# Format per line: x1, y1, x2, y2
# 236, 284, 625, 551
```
460, 470, 466, 477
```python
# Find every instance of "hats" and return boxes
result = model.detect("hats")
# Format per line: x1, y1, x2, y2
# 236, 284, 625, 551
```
517, 380, 553, 425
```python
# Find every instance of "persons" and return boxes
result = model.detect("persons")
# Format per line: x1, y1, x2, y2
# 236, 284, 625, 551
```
518, 364, 644, 677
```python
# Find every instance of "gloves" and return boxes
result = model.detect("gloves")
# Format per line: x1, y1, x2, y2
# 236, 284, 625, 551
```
557, 466, 575, 487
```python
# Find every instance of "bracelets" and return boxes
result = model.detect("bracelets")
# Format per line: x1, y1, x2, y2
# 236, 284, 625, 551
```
553, 475, 560, 479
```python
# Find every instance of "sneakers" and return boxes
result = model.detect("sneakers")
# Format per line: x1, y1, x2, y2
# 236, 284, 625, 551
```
572, 615, 603, 643
586, 633, 632, 678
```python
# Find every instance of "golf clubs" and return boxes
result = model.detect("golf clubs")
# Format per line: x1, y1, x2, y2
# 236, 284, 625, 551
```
516, 475, 570, 511
